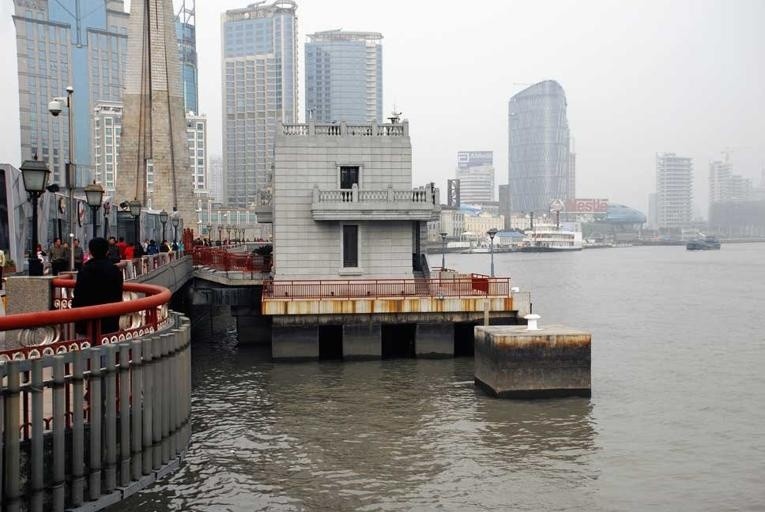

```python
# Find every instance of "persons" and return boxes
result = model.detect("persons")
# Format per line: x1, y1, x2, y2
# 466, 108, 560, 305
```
36, 235, 184, 280
71, 237, 124, 401
192, 237, 209, 247
0, 248, 5, 291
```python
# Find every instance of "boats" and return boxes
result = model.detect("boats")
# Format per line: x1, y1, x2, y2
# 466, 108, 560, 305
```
687, 239, 720, 250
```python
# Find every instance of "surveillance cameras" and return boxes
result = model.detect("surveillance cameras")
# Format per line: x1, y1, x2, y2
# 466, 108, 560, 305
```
48, 101, 62, 116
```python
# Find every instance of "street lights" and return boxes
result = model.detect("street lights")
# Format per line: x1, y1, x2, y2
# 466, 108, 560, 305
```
18, 150, 51, 276
159, 208, 168, 243
439, 231, 448, 272
171, 218, 180, 244
84, 179, 105, 240
487, 227, 498, 277
207, 223, 245, 247
128, 198, 142, 258
